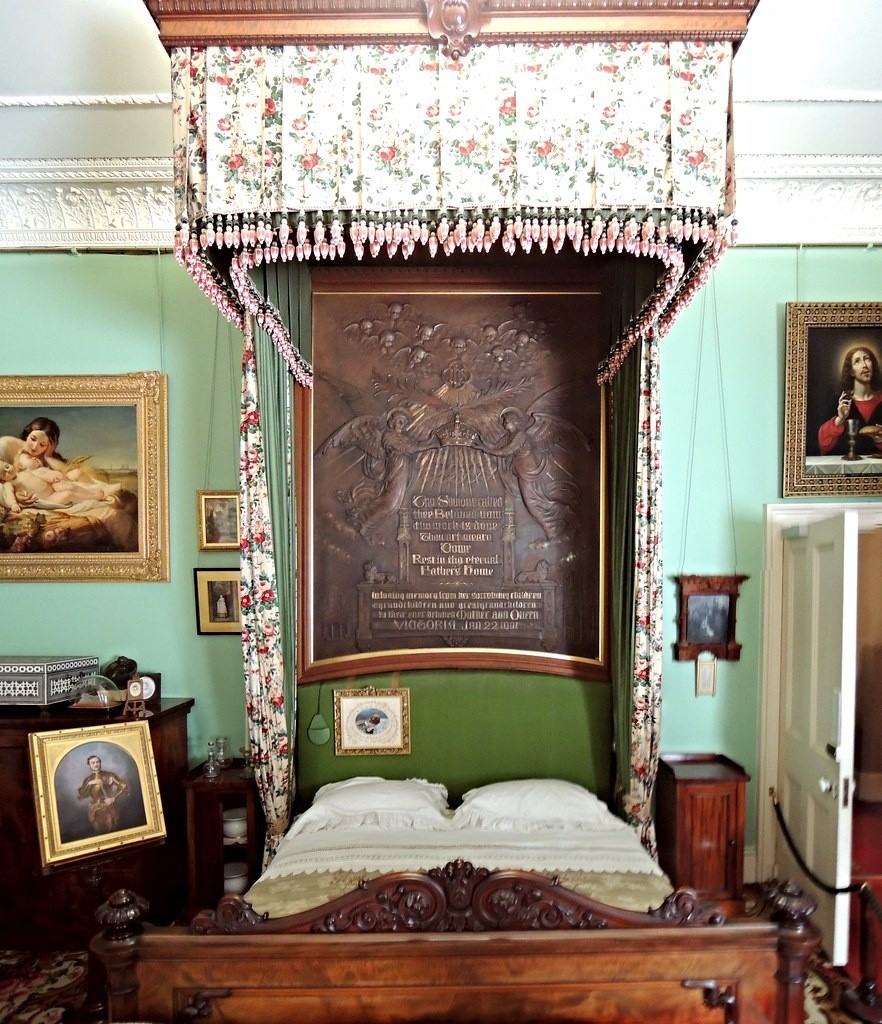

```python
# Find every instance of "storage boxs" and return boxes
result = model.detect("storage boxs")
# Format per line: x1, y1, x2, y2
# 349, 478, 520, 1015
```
0, 655, 100, 706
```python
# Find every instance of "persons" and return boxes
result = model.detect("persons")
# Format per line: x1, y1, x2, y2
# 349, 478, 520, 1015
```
472, 383, 591, 551
344, 304, 546, 372
313, 411, 441, 547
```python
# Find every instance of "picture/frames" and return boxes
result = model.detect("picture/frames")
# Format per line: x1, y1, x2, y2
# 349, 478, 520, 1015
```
196, 490, 240, 552
193, 567, 242, 636
332, 687, 412, 757
0, 373, 170, 584
294, 267, 608, 685
27, 719, 168, 870
783, 301, 882, 499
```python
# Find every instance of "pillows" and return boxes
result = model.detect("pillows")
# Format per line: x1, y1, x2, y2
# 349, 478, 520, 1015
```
452, 778, 628, 834
284, 775, 451, 840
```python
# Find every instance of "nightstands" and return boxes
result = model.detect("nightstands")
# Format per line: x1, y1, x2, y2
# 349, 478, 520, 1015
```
655, 752, 752, 917
179, 758, 258, 921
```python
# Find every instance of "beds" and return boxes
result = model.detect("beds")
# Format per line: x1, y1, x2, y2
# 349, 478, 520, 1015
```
89, 0, 823, 1024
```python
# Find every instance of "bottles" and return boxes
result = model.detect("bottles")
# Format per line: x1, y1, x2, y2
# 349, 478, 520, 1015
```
204, 737, 220, 778
214, 737, 232, 768
